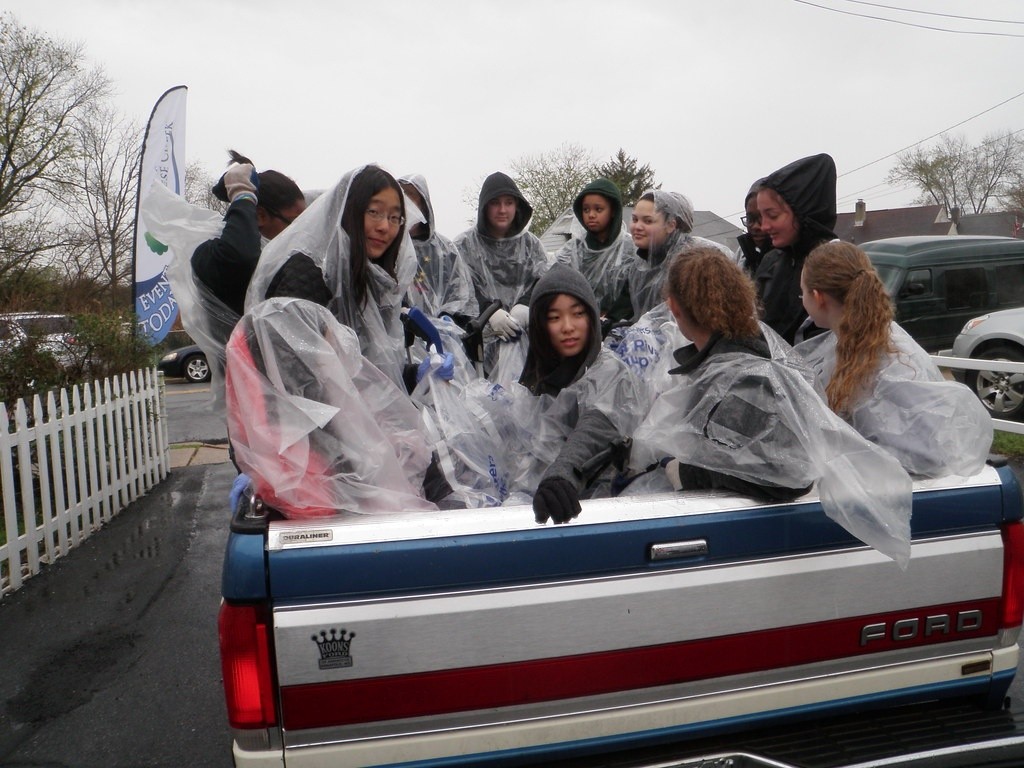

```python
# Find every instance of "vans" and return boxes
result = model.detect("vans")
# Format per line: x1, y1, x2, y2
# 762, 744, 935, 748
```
849, 232, 1024, 354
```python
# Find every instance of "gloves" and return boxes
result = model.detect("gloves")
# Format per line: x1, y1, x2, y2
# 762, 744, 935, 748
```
489, 309, 521, 342
224, 162, 259, 205
510, 304, 531, 333
533, 476, 581, 525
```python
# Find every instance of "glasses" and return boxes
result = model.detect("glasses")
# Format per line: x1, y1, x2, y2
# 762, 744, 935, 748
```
741, 216, 760, 227
366, 208, 408, 226
269, 211, 291, 226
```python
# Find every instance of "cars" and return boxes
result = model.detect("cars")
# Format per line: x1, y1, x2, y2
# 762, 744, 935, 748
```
937, 306, 1024, 420
0, 311, 92, 373
157, 343, 213, 384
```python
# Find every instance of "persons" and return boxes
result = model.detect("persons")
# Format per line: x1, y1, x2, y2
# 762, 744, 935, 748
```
481, 263, 634, 523
400, 173, 480, 360
598, 189, 726, 330
753, 152, 837, 345
551, 179, 636, 315
735, 177, 774, 279
247, 162, 454, 397
190, 150, 303, 376
225, 299, 467, 512
605, 245, 813, 504
456, 172, 550, 383
797, 241, 995, 478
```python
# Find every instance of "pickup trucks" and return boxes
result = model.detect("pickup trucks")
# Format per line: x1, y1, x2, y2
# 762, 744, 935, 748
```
214, 398, 1024, 768
